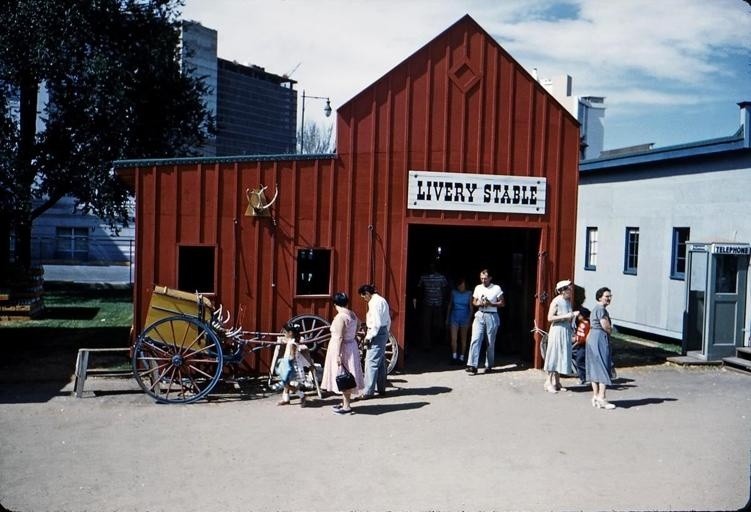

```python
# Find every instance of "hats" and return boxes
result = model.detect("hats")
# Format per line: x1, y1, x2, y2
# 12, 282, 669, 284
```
556, 280, 571, 289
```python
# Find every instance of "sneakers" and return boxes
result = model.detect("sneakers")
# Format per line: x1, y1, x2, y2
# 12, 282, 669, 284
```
453, 357, 491, 373
278, 394, 306, 407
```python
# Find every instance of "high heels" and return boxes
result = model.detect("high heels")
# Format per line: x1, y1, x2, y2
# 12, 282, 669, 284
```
544, 384, 567, 393
592, 396, 616, 410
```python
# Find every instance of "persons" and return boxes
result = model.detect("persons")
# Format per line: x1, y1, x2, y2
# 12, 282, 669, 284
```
354, 285, 392, 399
414, 262, 448, 356
277, 322, 306, 408
465, 268, 505, 374
542, 280, 580, 393
444, 278, 473, 362
584, 287, 616, 409
319, 293, 364, 414
572, 307, 591, 384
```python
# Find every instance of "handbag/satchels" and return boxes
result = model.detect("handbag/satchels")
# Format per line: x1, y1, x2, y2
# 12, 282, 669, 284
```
336, 372, 357, 391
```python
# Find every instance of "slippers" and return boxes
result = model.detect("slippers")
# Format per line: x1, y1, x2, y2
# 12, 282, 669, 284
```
333, 405, 352, 413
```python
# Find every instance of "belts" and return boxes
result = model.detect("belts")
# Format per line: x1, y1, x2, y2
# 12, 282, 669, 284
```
481, 310, 495, 314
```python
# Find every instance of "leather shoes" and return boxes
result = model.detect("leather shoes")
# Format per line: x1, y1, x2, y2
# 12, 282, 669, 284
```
355, 393, 385, 400
